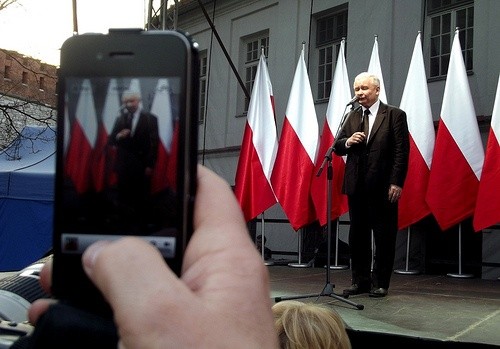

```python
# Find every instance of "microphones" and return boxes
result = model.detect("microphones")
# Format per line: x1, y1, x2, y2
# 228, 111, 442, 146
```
347, 94, 360, 106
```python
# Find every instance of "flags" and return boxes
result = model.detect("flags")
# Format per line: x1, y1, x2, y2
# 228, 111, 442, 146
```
473, 74, 500, 233
270, 41, 320, 232
367, 35, 388, 105
64, 77, 178, 193
310, 37, 353, 227
234, 46, 279, 223
398, 31, 436, 230
425, 27, 486, 231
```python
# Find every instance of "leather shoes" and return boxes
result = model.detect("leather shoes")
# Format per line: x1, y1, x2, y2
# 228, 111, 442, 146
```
343, 284, 370, 295
369, 286, 387, 297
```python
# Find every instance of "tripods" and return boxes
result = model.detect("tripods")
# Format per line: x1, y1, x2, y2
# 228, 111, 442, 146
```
274, 106, 367, 309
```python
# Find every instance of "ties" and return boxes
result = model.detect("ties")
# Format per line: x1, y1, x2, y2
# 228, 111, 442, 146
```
129, 113, 133, 133
361, 110, 370, 154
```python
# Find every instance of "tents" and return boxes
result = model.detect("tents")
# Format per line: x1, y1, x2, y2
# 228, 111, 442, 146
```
0, 125, 57, 271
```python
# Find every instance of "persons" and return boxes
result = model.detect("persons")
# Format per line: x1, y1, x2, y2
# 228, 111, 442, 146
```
28, 162, 279, 349
271, 300, 352, 349
257, 235, 271, 260
333, 72, 410, 297
108, 90, 159, 230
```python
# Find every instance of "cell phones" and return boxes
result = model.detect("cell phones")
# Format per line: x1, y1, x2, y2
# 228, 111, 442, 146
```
27, 27, 202, 349
